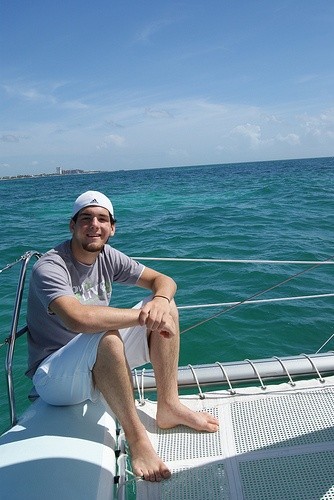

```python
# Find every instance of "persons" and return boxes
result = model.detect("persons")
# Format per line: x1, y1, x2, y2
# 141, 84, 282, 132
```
25, 190, 219, 482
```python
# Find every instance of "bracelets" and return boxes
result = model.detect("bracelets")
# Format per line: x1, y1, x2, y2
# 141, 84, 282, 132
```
151, 295, 170, 304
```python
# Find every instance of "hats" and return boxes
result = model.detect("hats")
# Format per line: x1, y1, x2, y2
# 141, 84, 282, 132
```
71, 189, 115, 218
